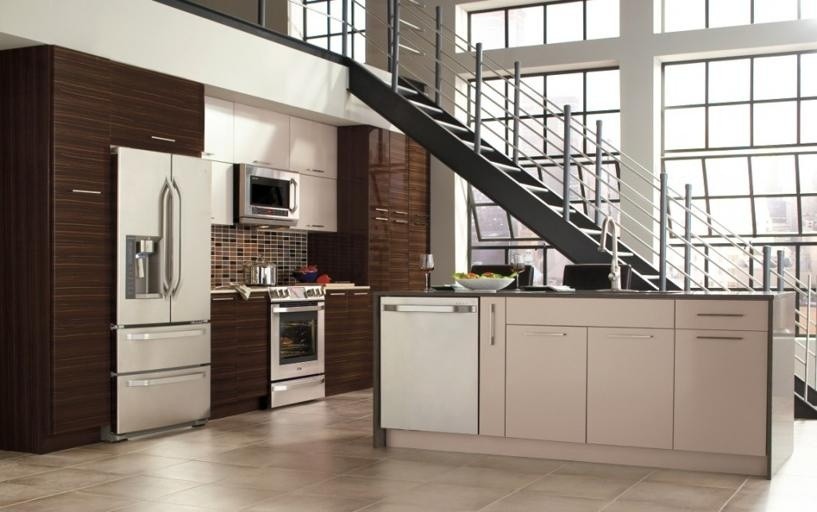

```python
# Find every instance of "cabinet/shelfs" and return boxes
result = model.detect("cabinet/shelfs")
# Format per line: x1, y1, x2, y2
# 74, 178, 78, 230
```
379, 297, 769, 478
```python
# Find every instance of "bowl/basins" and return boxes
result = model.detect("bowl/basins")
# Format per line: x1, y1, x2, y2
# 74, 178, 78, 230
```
291, 270, 319, 282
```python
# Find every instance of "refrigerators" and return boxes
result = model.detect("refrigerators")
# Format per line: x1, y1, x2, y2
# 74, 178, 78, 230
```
108, 143, 214, 443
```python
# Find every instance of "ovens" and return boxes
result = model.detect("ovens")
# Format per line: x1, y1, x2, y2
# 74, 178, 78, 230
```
270, 302, 327, 408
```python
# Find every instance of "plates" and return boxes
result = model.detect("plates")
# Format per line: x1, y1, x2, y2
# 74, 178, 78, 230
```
457, 277, 517, 293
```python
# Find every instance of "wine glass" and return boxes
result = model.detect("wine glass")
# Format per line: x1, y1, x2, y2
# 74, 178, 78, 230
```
506, 250, 528, 292
417, 252, 437, 292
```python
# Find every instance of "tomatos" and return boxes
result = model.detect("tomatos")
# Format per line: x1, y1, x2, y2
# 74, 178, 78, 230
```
297, 264, 318, 273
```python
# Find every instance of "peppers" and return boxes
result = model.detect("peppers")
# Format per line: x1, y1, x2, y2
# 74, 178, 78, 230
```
316, 273, 331, 283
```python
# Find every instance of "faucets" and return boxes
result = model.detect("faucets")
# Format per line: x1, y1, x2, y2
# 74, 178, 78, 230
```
597, 218, 621, 288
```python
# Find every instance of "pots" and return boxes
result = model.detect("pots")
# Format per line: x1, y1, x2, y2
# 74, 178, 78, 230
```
242, 258, 279, 287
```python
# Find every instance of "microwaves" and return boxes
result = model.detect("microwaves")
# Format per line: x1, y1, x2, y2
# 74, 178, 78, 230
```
232, 162, 302, 227
